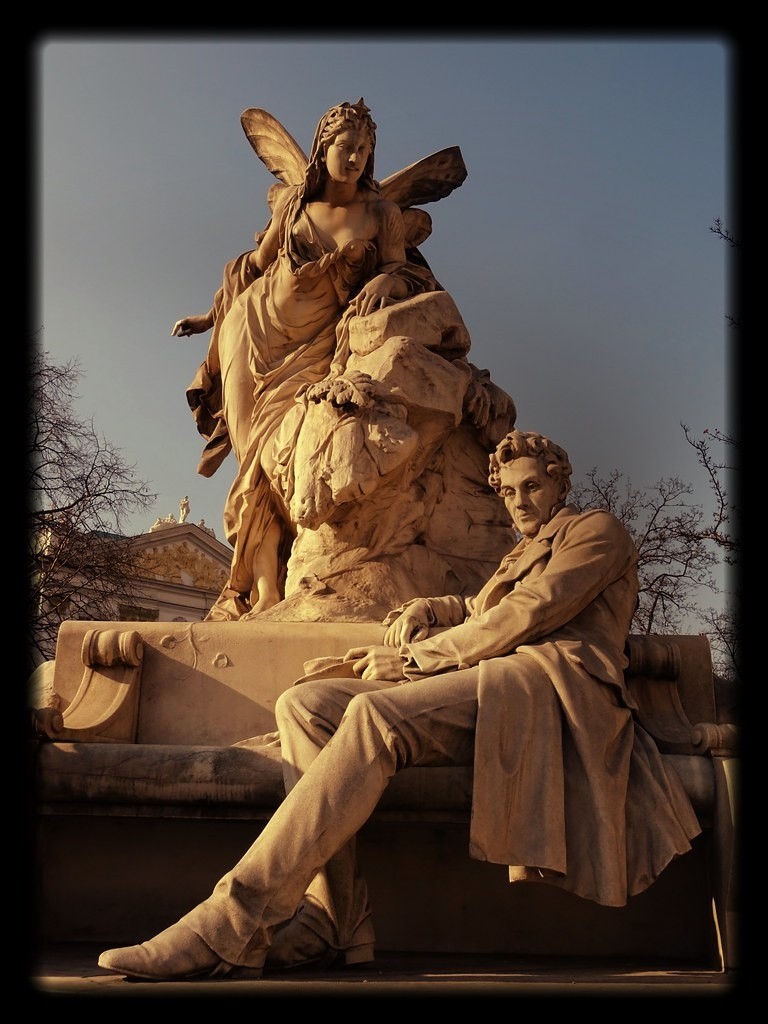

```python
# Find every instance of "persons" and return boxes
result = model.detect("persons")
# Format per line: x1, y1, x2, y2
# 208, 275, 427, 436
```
97, 431, 703, 981
171, 97, 408, 619
149, 495, 216, 541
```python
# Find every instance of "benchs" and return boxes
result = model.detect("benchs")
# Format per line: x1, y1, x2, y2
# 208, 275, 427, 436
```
31, 629, 736, 979
